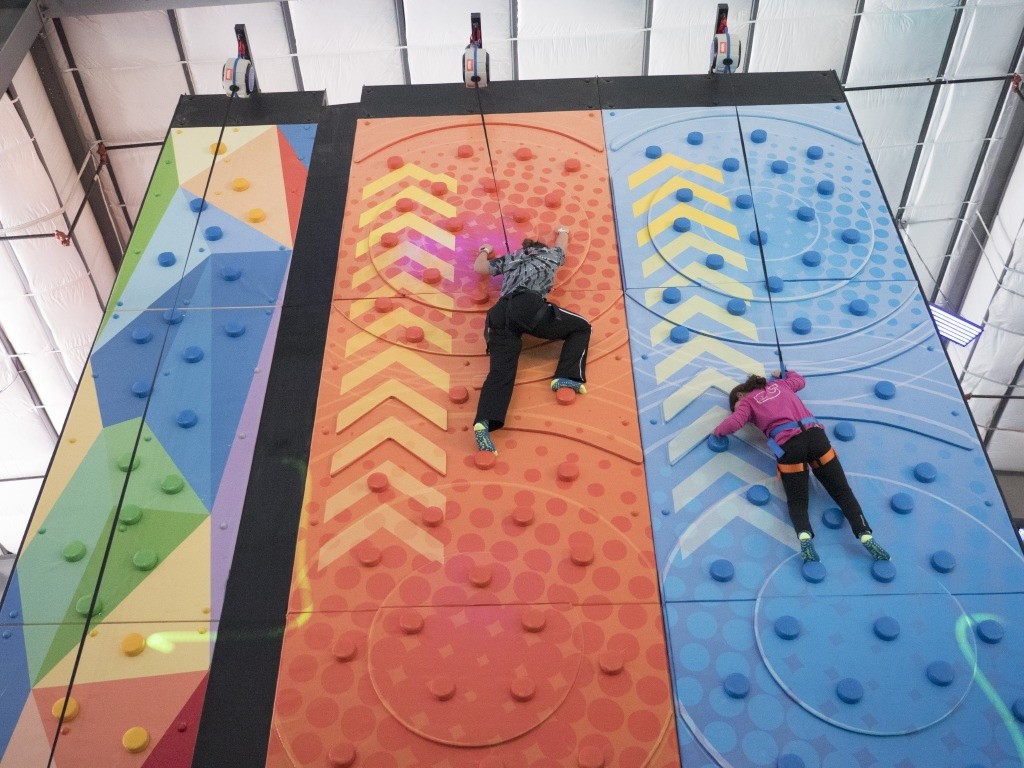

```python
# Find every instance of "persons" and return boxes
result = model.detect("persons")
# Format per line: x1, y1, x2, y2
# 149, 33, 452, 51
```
710, 369, 890, 560
473, 226, 592, 456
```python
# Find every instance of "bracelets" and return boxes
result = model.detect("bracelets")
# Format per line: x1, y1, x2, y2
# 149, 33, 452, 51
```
558, 228, 569, 233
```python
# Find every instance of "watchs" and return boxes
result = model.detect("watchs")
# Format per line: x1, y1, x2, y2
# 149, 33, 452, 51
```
479, 247, 489, 254
